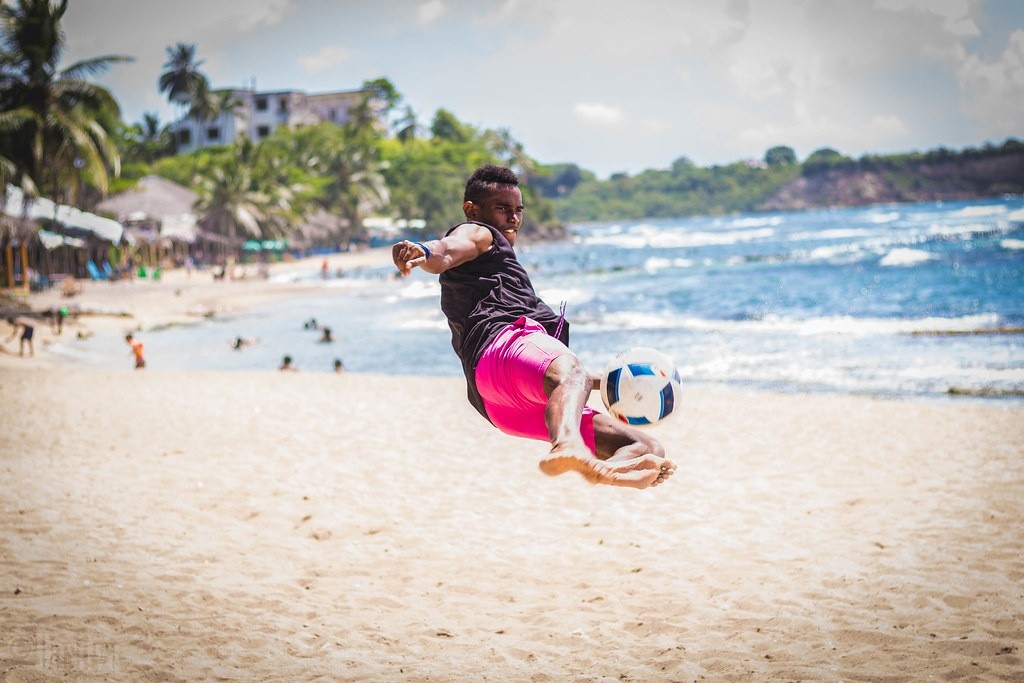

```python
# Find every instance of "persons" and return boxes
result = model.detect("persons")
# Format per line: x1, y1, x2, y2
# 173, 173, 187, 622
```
3, 316, 37, 360
44, 243, 405, 353
334, 359, 346, 372
391, 165, 678, 489
125, 334, 146, 368
279, 356, 300, 371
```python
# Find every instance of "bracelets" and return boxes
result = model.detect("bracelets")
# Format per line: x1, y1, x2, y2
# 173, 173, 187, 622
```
414, 243, 431, 260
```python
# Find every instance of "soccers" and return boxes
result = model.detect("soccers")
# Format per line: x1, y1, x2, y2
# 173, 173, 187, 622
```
600, 346, 682, 428
